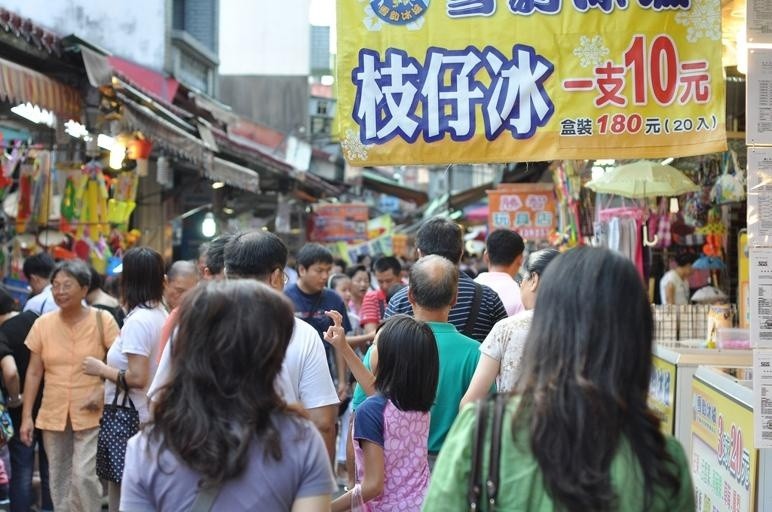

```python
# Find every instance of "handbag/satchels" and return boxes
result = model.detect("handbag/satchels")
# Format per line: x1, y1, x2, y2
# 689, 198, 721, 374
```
96, 405, 139, 483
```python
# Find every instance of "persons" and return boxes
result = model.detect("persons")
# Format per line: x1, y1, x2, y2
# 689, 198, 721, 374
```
1, 218, 698, 512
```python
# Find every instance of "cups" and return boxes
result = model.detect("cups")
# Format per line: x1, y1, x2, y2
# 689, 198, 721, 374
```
708, 303, 734, 345
715, 329, 748, 348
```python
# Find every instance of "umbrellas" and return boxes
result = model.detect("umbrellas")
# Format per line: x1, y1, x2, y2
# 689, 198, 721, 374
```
585, 160, 701, 247
